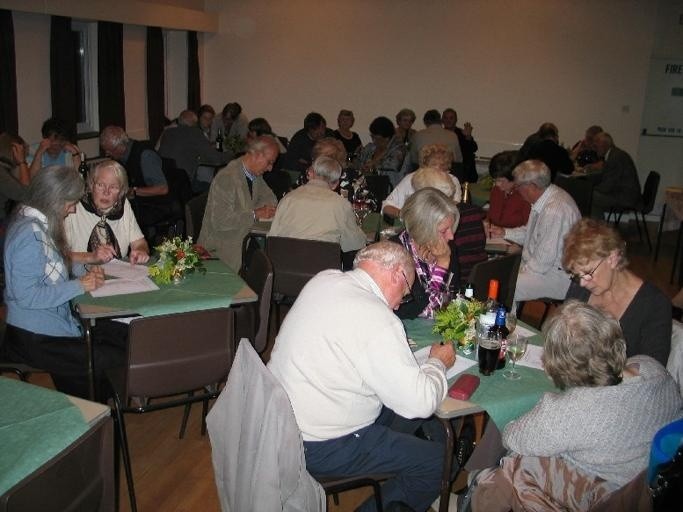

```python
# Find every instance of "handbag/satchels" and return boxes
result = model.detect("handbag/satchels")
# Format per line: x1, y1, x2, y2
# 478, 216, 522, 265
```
650, 446, 682, 512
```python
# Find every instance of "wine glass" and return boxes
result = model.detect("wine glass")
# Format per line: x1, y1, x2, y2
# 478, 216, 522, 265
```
504, 334, 527, 381
475, 307, 517, 354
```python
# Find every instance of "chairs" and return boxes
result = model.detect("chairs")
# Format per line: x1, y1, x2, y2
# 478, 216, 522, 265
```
240, 238, 274, 355
554, 165, 660, 250
113, 306, 236, 512
467, 154, 521, 314
162, 158, 215, 243
267, 235, 341, 336
240, 337, 383, 511
1, 372, 113, 511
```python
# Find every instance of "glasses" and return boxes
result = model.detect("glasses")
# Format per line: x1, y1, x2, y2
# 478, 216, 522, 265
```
402, 271, 413, 304
568, 260, 602, 283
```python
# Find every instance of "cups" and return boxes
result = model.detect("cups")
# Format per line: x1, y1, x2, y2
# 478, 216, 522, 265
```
477, 329, 500, 377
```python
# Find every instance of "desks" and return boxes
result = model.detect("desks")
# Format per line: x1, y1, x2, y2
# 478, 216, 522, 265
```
67, 243, 258, 401
654, 185, 683, 286
399, 310, 553, 510
249, 211, 404, 248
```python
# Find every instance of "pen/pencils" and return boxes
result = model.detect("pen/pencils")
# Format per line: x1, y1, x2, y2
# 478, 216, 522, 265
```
83, 264, 91, 273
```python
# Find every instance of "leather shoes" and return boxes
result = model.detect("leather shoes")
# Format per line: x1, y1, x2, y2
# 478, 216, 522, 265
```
453, 436, 473, 495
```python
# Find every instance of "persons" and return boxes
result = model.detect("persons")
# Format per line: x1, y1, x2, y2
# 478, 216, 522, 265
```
2, 119, 169, 405
266, 188, 683, 512
163, 99, 643, 354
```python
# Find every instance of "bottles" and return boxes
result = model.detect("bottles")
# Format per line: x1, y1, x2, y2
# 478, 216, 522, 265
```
403, 129, 410, 158
346, 151, 354, 181
289, 171, 305, 190
459, 182, 471, 217
478, 279, 502, 358
214, 128, 223, 153
76, 150, 88, 194
448, 281, 477, 354
488, 304, 509, 369
